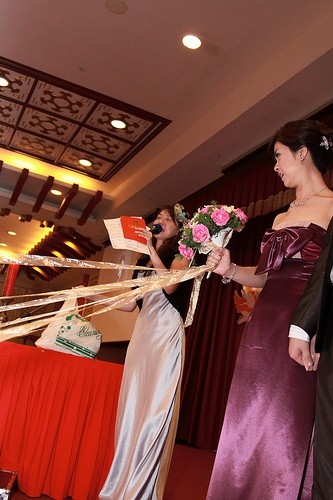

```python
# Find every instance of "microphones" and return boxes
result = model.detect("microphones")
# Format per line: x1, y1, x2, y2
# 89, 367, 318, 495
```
150, 224, 162, 234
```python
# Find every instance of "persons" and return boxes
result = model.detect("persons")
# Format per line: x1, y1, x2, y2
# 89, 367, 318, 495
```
206, 119, 333, 500
72, 206, 195, 500
287, 215, 333, 500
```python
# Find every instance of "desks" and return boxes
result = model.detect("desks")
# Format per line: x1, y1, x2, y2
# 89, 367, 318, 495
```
0, 340, 125, 500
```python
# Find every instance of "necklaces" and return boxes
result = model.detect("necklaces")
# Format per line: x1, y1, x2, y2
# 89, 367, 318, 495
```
289, 187, 329, 208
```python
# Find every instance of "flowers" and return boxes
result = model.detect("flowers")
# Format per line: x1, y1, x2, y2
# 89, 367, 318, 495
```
174, 200, 248, 279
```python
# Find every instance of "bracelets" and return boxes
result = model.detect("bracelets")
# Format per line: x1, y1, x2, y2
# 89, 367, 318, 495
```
221, 263, 237, 284
